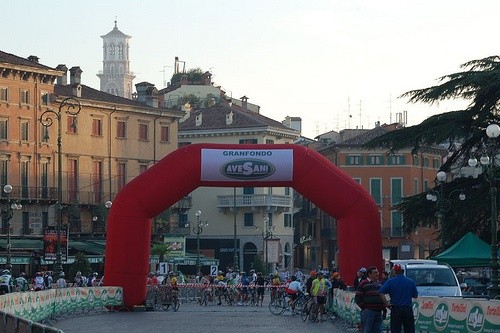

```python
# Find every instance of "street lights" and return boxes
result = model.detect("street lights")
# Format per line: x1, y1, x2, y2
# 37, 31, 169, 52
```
468, 127, 500, 299
3, 185, 22, 270
426, 171, 465, 253
185, 210, 209, 274
40, 98, 81, 281
255, 217, 275, 275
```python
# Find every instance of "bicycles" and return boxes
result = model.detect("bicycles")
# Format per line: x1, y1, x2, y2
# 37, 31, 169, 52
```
268, 282, 321, 323
148, 283, 180, 313
199, 275, 261, 308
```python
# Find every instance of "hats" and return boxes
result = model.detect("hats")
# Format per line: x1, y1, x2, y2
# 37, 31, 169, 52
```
359, 268, 367, 273
392, 264, 401, 270
310, 271, 317, 276
46, 272, 50, 275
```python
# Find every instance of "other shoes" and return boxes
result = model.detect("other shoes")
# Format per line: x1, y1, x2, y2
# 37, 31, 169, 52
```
218, 301, 263, 307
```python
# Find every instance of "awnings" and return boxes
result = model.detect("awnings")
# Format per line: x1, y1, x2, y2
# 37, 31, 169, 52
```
0, 252, 220, 265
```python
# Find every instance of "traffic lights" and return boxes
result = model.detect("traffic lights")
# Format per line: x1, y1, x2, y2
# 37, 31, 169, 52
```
92, 201, 112, 244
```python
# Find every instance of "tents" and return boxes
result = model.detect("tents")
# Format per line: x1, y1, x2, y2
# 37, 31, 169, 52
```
428, 232, 493, 298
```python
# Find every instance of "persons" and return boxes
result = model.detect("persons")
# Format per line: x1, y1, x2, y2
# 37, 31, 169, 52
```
0, 263, 416, 333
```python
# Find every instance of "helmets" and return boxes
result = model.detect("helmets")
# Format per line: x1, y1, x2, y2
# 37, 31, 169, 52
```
169, 271, 175, 277
76, 271, 81, 275
19, 272, 25, 276
218, 271, 223, 274
148, 272, 154, 275
35, 272, 41, 276
3, 269, 10, 274
93, 272, 98, 276
